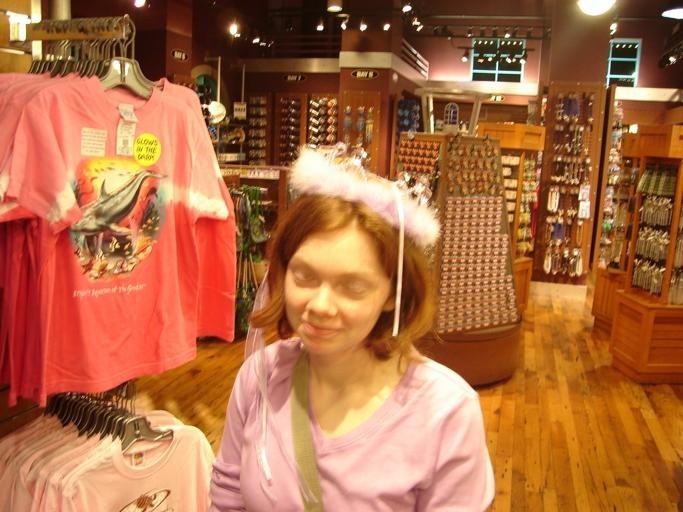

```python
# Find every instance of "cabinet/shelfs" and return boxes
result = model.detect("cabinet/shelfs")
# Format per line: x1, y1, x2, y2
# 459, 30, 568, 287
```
218, 164, 288, 260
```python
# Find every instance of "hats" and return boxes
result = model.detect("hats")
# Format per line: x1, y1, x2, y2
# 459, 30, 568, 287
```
203, 101, 227, 124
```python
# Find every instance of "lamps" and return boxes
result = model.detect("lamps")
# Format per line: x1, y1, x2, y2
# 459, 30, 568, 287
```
429, 24, 550, 38
455, 47, 535, 66
317, 0, 368, 32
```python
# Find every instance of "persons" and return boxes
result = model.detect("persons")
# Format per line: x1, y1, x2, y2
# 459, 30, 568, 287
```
208, 144, 496, 511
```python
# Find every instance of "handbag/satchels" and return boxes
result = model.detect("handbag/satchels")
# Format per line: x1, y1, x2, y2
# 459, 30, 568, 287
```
236, 215, 271, 254
235, 281, 258, 332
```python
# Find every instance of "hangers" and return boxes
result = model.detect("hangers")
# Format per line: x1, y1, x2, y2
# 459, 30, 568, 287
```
43, 381, 173, 454
28, 14, 162, 102
